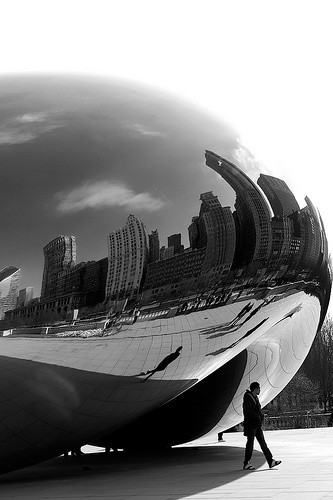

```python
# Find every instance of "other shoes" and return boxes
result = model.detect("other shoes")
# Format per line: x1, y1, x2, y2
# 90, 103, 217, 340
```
243, 464, 255, 470
269, 460, 281, 468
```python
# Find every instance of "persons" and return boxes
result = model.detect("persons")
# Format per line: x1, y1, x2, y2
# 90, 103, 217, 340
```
177, 286, 258, 328
130, 307, 142, 327
242, 382, 282, 472
218, 431, 227, 442
145, 346, 183, 381
